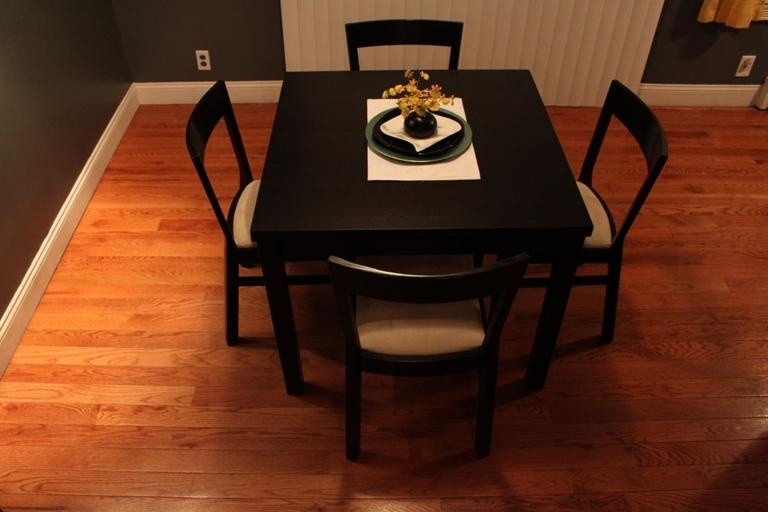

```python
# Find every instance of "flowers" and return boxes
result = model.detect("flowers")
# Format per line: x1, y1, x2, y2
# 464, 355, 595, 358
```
379, 68, 458, 114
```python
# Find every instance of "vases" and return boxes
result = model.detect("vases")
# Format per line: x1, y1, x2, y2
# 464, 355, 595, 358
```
401, 109, 438, 138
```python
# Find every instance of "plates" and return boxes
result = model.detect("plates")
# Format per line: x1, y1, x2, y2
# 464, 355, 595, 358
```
373, 106, 464, 157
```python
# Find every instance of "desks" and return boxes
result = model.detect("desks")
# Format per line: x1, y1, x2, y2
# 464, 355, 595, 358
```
251, 68, 594, 397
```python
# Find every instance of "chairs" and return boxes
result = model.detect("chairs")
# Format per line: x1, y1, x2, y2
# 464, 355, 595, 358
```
186, 79, 330, 348
344, 17, 466, 71
497, 77, 671, 345
329, 252, 531, 461
